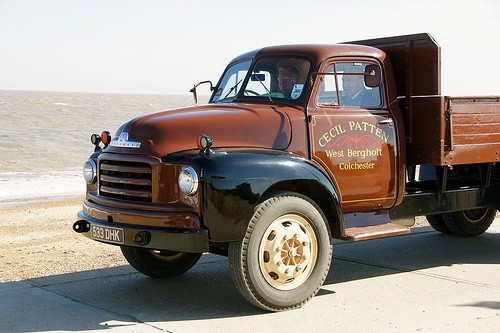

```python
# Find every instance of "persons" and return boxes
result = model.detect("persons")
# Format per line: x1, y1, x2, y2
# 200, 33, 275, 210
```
331, 68, 379, 106
277, 62, 299, 98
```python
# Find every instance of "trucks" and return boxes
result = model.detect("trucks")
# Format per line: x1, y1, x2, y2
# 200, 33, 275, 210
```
73, 32, 500, 313
